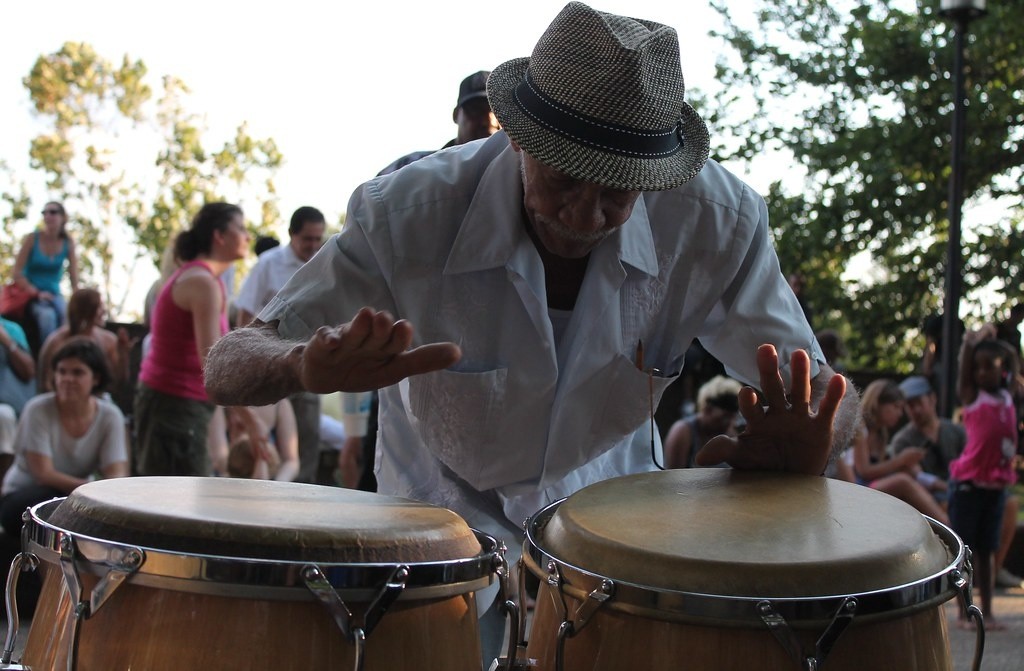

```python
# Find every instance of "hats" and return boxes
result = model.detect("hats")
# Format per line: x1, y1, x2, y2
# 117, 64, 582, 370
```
486, 1, 710, 191
457, 70, 490, 105
899, 377, 930, 399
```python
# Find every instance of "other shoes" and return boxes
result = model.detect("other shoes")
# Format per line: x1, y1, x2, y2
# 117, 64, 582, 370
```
985, 620, 1009, 631
956, 620, 976, 629
998, 569, 1024, 589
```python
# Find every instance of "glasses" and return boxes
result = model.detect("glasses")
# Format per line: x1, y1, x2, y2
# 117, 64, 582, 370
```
641, 367, 680, 470
42, 210, 61, 215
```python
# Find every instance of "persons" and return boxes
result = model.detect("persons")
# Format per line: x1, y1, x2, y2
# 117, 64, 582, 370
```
377, 68, 506, 181
0, 199, 1024, 631
201, 6, 863, 670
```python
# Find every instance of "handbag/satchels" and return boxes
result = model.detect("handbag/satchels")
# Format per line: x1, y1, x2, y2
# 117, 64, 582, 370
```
0, 283, 38, 318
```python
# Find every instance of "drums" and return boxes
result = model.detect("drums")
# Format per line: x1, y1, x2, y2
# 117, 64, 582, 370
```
520, 466, 987, 671
2, 474, 518, 671
